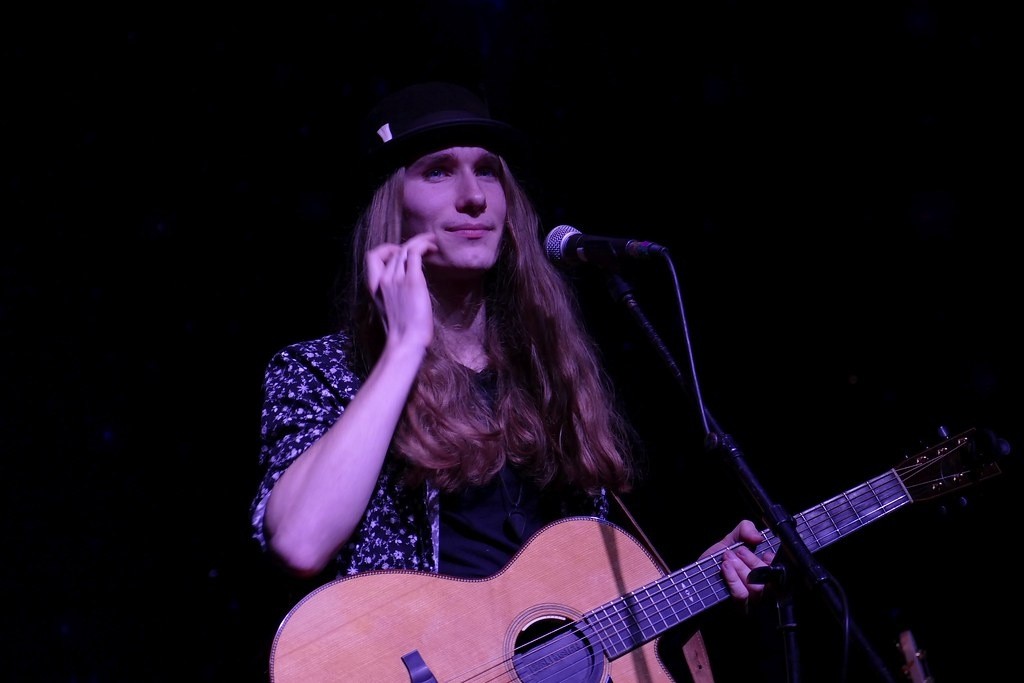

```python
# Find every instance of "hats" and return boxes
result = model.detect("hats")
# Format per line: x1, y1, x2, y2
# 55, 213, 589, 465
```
375, 81, 512, 176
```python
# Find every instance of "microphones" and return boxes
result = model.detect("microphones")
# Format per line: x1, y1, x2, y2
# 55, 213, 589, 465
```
541, 224, 668, 270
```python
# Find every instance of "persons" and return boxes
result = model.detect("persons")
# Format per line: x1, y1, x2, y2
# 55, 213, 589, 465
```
250, 82, 776, 610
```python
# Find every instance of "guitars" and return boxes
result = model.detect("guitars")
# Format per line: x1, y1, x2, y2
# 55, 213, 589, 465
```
267, 423, 1006, 683
894, 630, 931, 683
